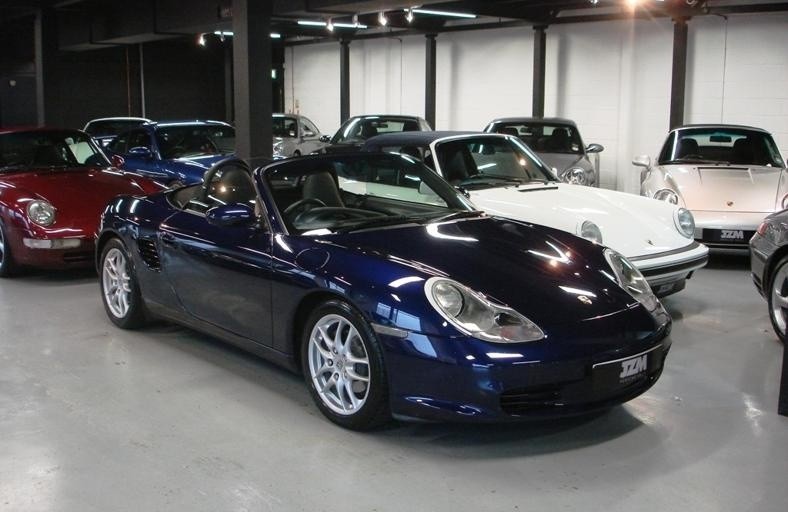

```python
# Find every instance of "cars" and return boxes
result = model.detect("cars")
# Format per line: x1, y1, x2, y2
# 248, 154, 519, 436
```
742, 208, 788, 343
628, 122, 788, 254
1, 123, 170, 272
65, 112, 152, 165
337, 126, 710, 302
217, 112, 329, 162
305, 114, 431, 160
84, 117, 235, 186
467, 115, 605, 187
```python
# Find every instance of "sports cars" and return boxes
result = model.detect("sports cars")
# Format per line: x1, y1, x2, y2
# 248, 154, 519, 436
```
89, 145, 673, 435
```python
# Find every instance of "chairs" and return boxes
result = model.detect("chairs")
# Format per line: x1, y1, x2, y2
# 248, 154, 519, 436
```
681, 138, 699, 155
498, 127, 519, 138
396, 148, 424, 188
732, 138, 745, 150
302, 171, 346, 218
402, 121, 419, 132
443, 150, 468, 180
365, 126, 377, 138
547, 129, 570, 153
